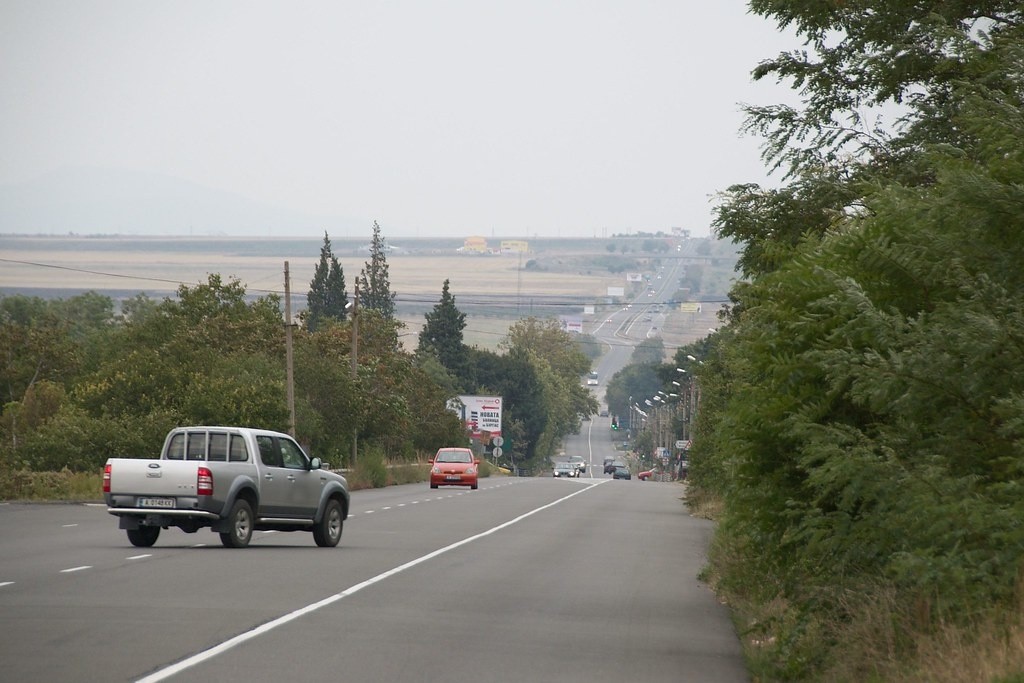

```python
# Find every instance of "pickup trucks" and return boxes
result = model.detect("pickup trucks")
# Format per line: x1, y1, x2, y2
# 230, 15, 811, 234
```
102, 424, 350, 550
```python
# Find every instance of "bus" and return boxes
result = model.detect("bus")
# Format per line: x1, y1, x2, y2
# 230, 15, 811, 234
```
587, 372, 599, 385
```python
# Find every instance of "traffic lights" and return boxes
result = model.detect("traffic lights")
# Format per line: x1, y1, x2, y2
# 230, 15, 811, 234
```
611, 423, 618, 430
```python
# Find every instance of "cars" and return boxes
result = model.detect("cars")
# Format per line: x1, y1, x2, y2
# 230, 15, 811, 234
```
429, 448, 481, 490
604, 456, 625, 476
600, 410, 609, 417
614, 468, 631, 480
568, 456, 586, 473
638, 466, 674, 482
552, 462, 580, 478
